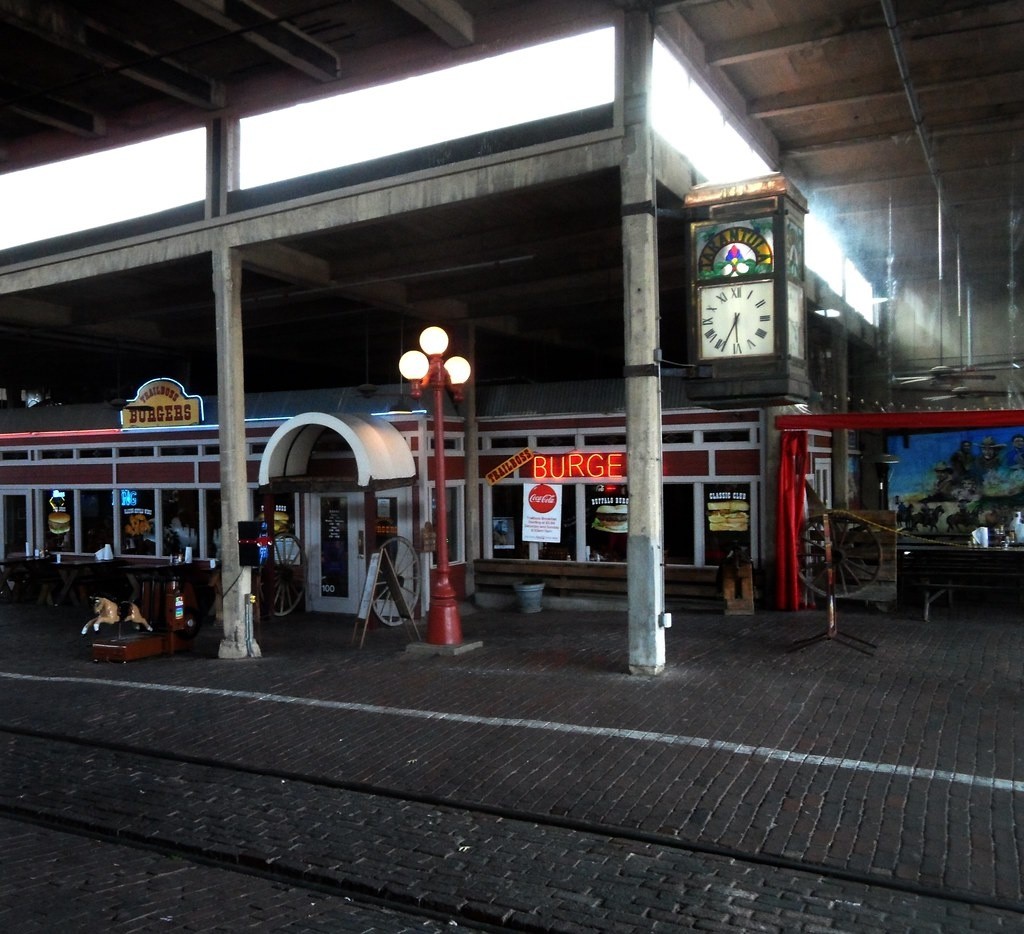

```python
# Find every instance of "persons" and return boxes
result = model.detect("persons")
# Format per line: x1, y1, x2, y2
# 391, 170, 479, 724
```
886, 427, 1024, 537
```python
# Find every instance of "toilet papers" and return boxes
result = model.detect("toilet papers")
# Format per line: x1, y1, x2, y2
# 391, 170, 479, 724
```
185, 547, 193, 565
94, 544, 114, 561
971, 526, 990, 548
25, 542, 31, 557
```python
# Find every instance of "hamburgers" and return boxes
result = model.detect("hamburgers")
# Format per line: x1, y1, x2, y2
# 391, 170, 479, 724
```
47, 513, 71, 534
592, 505, 627, 533
706, 500, 749, 531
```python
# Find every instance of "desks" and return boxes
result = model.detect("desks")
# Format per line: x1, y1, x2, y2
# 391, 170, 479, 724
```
1, 556, 261, 622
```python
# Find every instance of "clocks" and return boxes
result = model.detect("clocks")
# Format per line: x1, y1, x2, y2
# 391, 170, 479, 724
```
787, 279, 806, 361
696, 279, 776, 361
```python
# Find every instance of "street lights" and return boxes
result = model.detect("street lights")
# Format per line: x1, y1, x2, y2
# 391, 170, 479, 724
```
397, 323, 473, 646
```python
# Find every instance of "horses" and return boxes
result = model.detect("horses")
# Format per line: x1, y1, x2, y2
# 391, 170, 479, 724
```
913, 503, 946, 533
81, 597, 154, 635
896, 505, 913, 529
946, 505, 985, 532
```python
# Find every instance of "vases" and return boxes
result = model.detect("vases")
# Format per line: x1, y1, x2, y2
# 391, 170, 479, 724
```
513, 578, 546, 615
